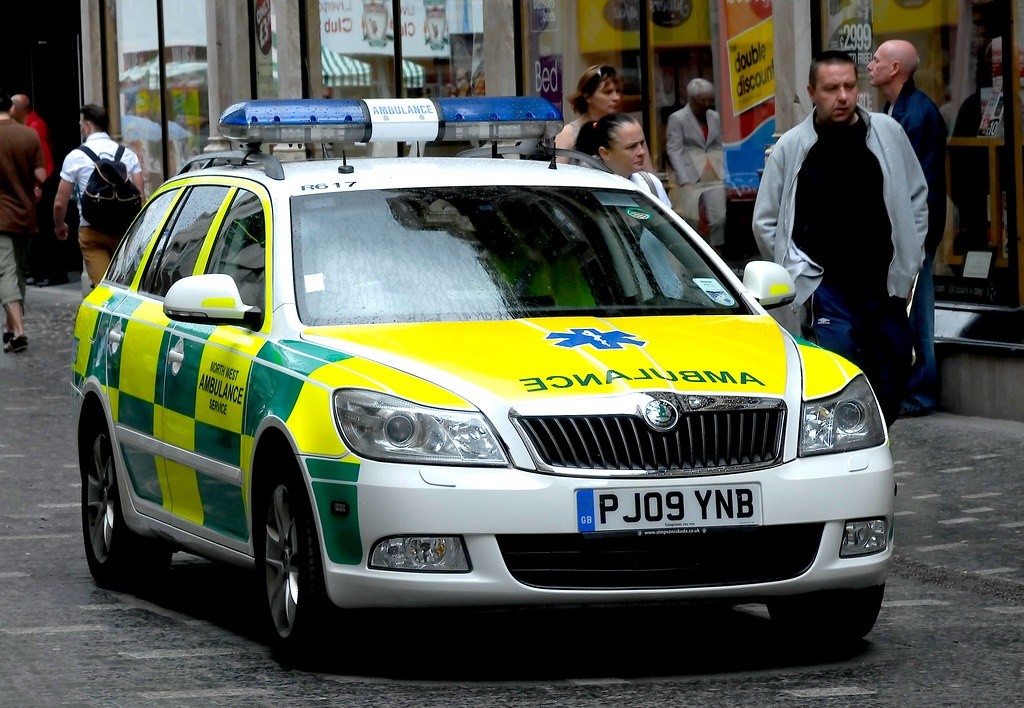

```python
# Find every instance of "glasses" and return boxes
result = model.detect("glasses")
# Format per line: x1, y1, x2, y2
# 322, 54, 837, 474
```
593, 66, 616, 81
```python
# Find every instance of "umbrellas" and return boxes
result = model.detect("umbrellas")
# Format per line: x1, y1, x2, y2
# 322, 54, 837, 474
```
121, 115, 194, 142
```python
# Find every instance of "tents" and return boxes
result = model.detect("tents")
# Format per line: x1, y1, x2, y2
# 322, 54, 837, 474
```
119, 44, 425, 98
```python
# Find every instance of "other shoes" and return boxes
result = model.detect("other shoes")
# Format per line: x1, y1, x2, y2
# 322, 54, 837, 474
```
25, 277, 41, 285
899, 398, 923, 417
37, 278, 68, 286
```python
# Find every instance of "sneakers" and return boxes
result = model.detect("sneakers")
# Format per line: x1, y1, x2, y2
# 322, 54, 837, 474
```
3, 327, 28, 352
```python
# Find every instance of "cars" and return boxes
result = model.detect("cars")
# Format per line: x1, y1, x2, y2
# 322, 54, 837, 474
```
71, 94, 898, 647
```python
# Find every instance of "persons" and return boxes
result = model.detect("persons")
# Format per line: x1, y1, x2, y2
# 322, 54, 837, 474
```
865, 38, 948, 418
665, 77, 727, 256
751, 51, 928, 428
576, 112, 694, 300
0, 93, 47, 355
52, 102, 145, 288
554, 62, 651, 169
10, 94, 69, 288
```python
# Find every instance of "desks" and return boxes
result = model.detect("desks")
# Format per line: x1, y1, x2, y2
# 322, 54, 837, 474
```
943, 135, 1008, 267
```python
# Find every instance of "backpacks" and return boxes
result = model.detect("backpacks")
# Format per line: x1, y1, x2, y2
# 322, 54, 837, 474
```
75, 146, 141, 235
